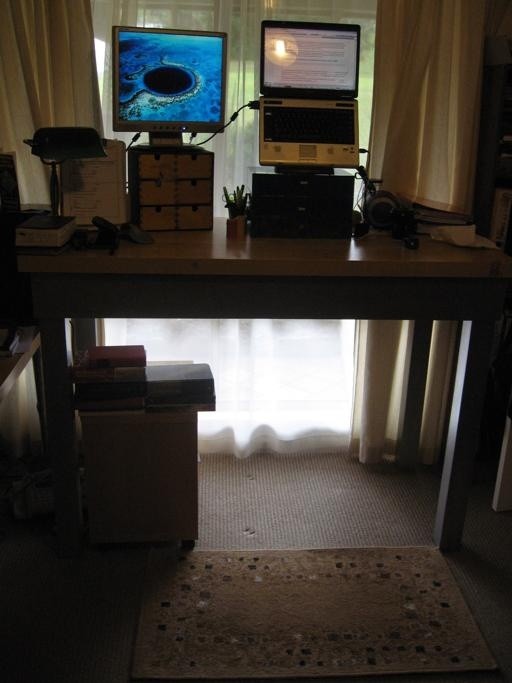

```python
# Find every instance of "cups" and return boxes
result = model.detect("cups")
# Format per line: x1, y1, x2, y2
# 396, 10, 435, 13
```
229, 208, 244, 219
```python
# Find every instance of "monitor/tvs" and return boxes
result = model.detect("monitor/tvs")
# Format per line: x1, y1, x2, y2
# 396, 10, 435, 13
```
112, 25, 228, 149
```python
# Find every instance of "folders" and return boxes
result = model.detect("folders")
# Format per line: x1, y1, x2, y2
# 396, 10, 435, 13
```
399, 189, 474, 236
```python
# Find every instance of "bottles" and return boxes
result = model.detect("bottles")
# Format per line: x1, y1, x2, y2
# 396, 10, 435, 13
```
226, 215, 246, 241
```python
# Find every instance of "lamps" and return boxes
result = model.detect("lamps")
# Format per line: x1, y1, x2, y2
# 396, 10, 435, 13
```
23, 127, 109, 218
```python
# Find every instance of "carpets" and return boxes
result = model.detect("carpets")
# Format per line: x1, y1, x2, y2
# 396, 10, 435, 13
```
129, 545, 499, 680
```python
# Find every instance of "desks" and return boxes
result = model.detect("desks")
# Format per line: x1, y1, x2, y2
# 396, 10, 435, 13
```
17, 217, 512, 551
0, 325, 51, 448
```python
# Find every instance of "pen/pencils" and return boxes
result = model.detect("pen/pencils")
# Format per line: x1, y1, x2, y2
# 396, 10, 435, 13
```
224, 186, 247, 205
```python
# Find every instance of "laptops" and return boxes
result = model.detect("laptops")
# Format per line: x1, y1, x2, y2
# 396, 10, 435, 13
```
260, 21, 361, 168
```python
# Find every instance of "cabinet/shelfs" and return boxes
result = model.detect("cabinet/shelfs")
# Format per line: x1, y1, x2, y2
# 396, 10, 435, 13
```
78, 412, 198, 549
